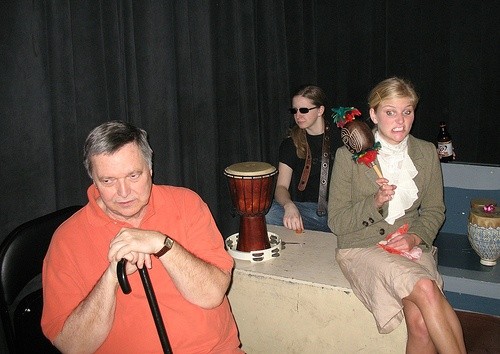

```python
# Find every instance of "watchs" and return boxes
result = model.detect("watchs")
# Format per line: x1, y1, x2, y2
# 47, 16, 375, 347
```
154, 235, 173, 258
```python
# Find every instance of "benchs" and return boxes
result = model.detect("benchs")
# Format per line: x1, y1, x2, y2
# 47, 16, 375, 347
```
226, 223, 438, 354
435, 163, 500, 318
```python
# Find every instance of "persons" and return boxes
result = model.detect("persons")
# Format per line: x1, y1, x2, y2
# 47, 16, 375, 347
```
328, 77, 466, 354
264, 85, 344, 234
41, 120, 246, 354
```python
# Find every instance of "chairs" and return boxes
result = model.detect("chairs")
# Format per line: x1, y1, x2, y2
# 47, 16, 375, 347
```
0, 205, 87, 354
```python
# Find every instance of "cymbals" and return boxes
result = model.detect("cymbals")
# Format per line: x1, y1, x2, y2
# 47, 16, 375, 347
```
224, 230, 286, 263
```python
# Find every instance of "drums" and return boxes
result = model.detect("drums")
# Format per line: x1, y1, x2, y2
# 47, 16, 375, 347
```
466, 198, 500, 266
221, 161, 280, 252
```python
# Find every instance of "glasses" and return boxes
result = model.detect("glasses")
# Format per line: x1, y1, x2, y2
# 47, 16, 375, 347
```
289, 107, 319, 115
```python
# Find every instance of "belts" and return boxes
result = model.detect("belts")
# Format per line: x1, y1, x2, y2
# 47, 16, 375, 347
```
298, 120, 330, 217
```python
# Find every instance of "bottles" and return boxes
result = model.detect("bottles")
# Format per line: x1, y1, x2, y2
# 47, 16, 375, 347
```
437, 121, 454, 163
